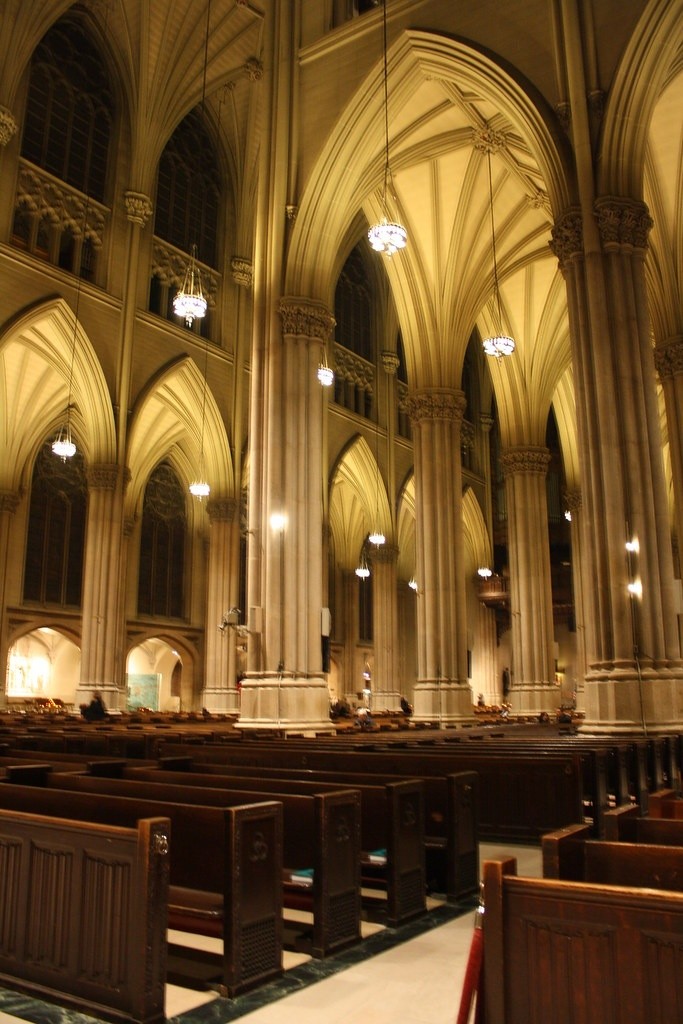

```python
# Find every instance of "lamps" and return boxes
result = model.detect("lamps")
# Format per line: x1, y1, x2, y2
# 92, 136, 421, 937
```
367, 0, 409, 260
483, 150, 515, 365
189, 336, 211, 503
174, 0, 210, 328
368, 421, 385, 551
50, 187, 89, 464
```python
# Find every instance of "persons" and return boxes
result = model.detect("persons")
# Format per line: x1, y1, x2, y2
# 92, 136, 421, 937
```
400, 697, 412, 715
555, 708, 571, 724
354, 707, 370, 725
539, 712, 549, 724
79, 690, 106, 719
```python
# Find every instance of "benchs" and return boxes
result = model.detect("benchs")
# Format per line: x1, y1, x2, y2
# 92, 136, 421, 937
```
0, 708, 683, 1024
